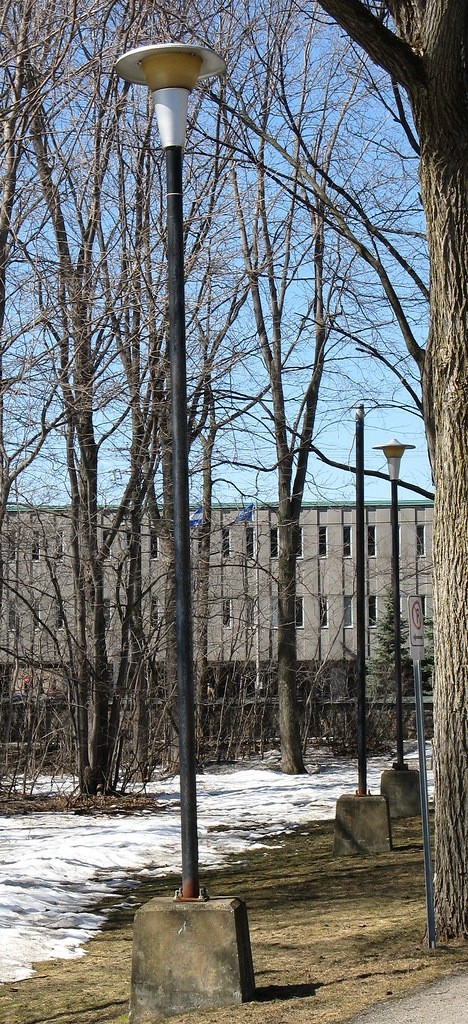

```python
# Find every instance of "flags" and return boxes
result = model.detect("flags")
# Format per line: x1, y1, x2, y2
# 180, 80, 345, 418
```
236, 503, 253, 520
189, 504, 203, 528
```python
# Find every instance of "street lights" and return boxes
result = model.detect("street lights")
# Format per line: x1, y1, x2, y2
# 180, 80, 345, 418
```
112, 44, 229, 901
374, 437, 417, 770
